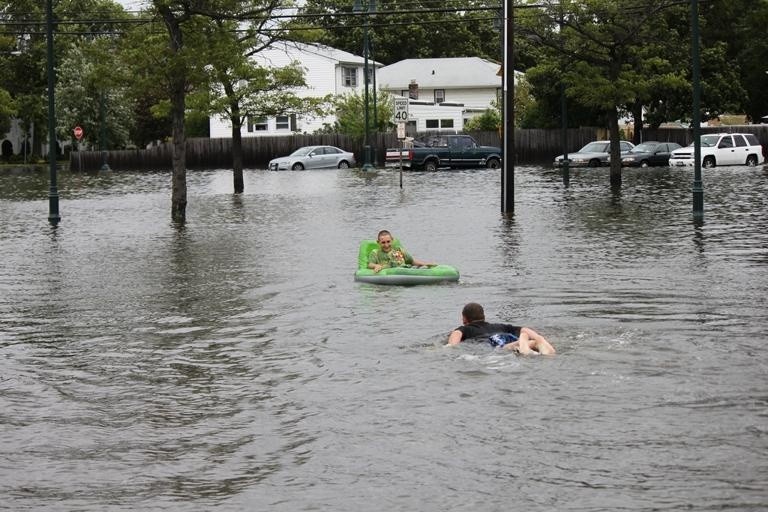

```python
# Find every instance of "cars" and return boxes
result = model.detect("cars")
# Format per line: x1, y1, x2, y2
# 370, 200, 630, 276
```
608, 140, 684, 169
264, 143, 356, 172
547, 138, 636, 168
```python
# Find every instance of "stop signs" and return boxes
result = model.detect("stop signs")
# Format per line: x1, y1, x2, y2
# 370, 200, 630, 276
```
73, 126, 84, 140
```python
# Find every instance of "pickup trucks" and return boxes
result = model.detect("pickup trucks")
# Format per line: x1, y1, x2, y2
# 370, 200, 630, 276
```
385, 132, 501, 170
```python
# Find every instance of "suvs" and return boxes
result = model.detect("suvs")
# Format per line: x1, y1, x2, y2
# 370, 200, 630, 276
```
667, 132, 766, 167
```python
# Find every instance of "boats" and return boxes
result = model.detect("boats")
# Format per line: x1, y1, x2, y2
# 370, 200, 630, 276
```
353, 236, 463, 286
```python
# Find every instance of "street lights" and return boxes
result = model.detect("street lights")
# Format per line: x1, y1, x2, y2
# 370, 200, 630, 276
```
351, 1, 380, 176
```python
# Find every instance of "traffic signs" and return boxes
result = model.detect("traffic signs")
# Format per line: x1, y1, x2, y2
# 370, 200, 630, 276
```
394, 97, 410, 124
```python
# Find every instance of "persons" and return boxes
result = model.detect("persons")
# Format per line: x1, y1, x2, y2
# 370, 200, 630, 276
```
445, 302, 554, 359
366, 230, 438, 273
